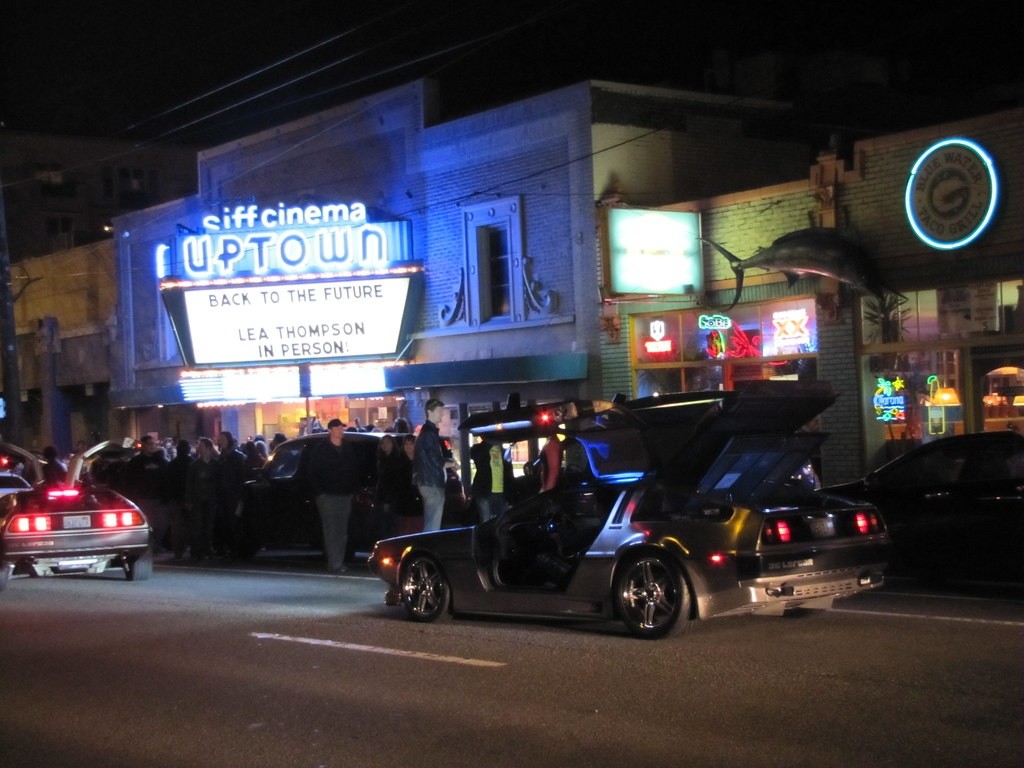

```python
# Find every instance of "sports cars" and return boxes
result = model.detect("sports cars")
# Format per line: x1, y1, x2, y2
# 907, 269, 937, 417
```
1, 437, 154, 584
364, 378, 890, 641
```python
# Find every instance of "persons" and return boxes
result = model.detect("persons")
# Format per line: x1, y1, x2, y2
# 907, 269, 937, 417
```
43, 430, 287, 567
538, 435, 564, 494
415, 399, 456, 533
470, 430, 514, 521
312, 415, 424, 574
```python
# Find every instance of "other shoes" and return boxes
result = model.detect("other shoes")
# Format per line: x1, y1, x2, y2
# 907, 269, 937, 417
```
337, 566, 350, 575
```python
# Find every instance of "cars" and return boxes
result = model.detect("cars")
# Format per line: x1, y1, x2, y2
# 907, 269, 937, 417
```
811, 430, 1024, 575
260, 431, 466, 544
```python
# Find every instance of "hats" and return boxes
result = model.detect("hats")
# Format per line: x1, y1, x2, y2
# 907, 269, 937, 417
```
328, 418, 347, 428
42, 446, 58, 457
